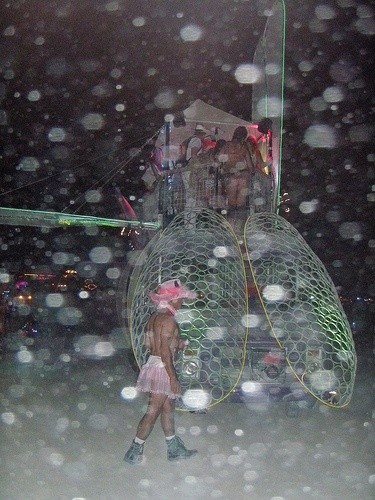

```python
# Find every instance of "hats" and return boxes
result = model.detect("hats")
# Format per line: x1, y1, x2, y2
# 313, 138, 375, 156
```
147, 278, 199, 303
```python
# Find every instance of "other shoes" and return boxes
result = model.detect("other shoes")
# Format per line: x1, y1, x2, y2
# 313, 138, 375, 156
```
122, 439, 146, 465
166, 435, 198, 462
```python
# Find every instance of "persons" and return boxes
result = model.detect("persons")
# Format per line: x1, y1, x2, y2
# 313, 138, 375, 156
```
181, 123, 271, 234
124, 279, 199, 465
134, 137, 163, 222
